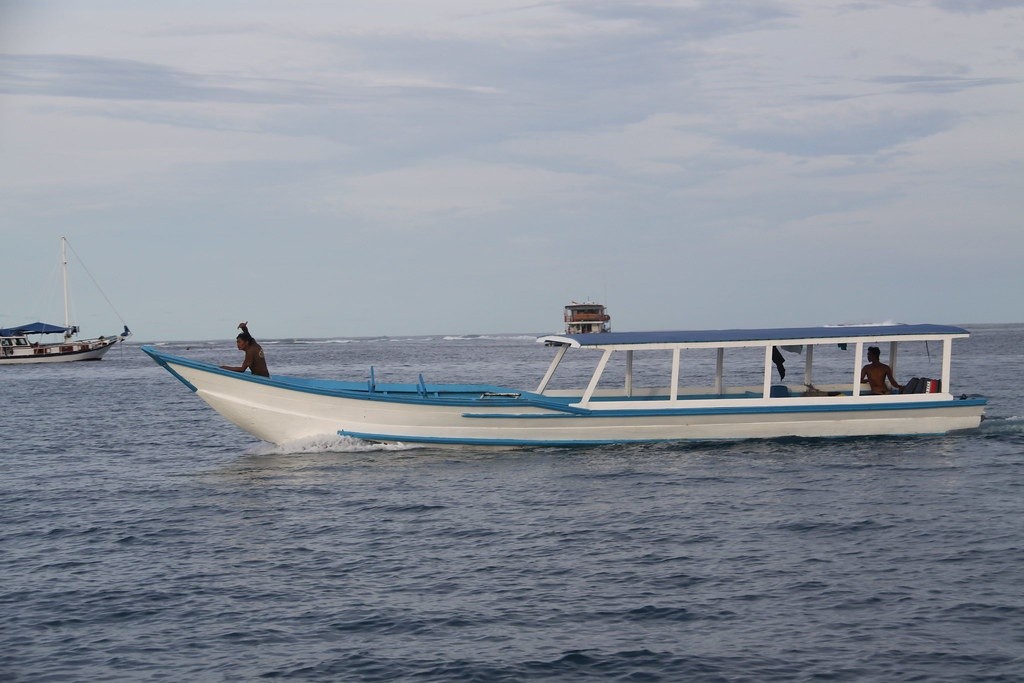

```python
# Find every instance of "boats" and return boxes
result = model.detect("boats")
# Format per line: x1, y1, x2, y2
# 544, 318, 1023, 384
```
139, 323, 989, 449
545, 296, 611, 346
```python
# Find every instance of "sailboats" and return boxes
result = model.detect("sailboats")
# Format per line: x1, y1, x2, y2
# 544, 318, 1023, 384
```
0, 236, 134, 364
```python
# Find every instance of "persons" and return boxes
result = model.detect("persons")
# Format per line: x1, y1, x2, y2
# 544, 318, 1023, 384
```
219, 321, 269, 377
861, 347, 902, 395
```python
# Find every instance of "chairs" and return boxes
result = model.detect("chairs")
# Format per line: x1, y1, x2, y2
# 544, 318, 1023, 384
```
770, 385, 789, 397
